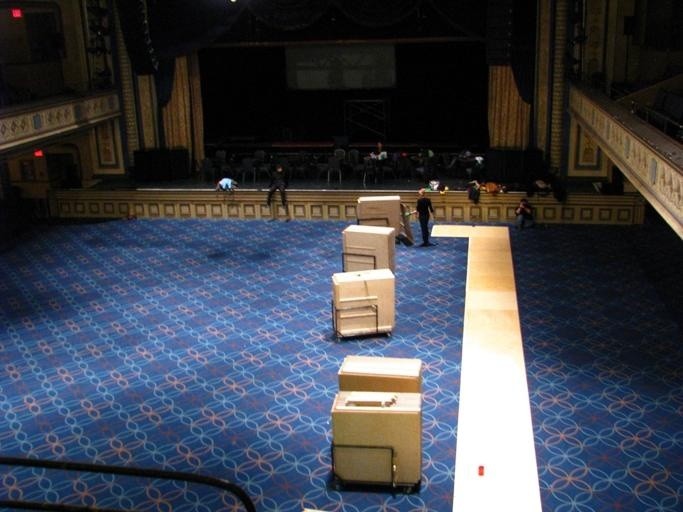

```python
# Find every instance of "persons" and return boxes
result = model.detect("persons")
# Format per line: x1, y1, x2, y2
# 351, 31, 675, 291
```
401, 188, 435, 246
514, 198, 533, 229
215, 177, 239, 192
267, 166, 288, 207
